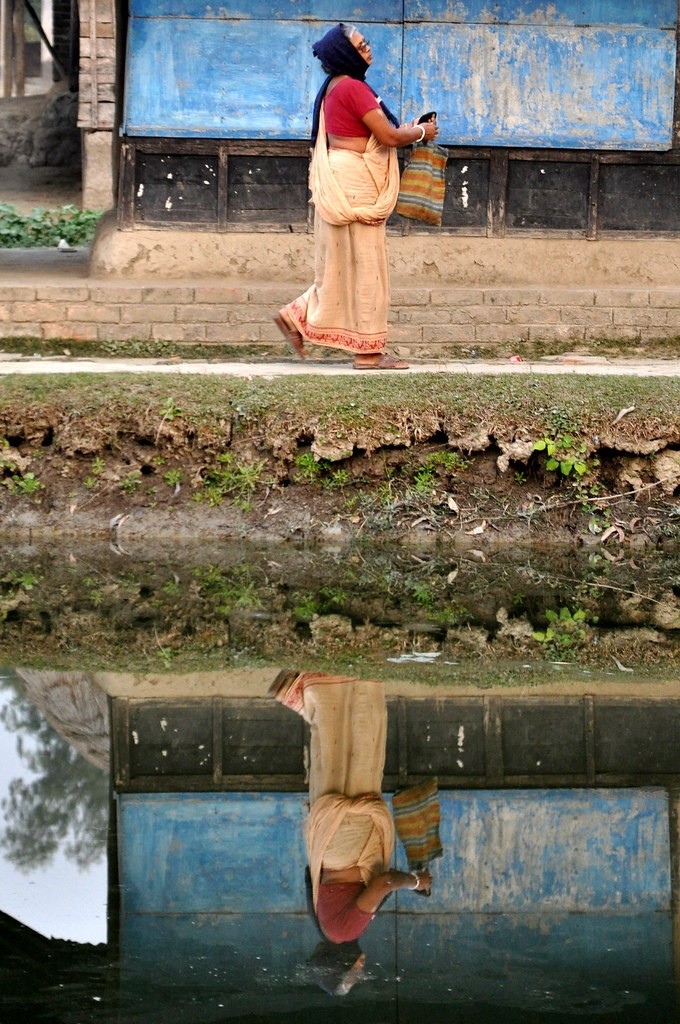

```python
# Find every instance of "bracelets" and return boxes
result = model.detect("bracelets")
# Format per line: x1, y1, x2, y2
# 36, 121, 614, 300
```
408, 873, 420, 890
404, 122, 408, 130
412, 124, 426, 143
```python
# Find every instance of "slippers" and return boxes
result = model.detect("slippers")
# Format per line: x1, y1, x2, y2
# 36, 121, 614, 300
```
273, 316, 305, 361
353, 353, 409, 369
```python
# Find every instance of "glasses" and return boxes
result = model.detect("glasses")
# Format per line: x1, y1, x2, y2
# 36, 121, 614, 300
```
356, 40, 370, 52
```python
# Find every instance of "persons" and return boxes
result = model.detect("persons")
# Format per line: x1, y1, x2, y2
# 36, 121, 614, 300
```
273, 18, 438, 369
266, 670, 431, 998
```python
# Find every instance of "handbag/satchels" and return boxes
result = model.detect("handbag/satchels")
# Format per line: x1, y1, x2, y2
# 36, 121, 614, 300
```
396, 111, 449, 227
